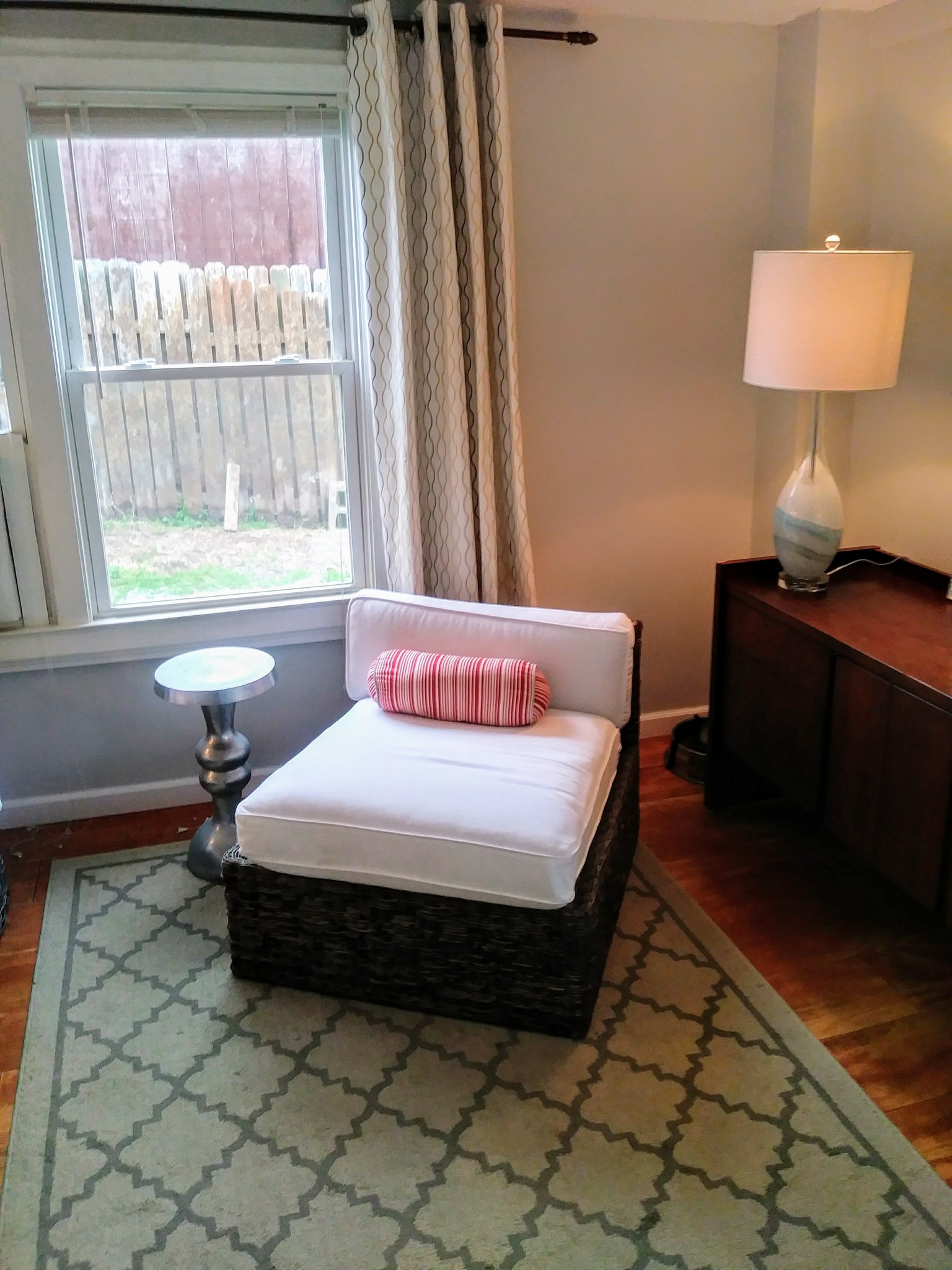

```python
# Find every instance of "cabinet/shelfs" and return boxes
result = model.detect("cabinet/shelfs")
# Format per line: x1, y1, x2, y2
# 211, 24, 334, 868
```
703, 545, 952, 927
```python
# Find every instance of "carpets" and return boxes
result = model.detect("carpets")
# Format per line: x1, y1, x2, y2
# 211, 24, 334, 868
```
0, 838, 952, 1270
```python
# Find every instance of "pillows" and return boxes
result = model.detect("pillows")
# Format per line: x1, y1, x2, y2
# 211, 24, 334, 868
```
365, 645, 549, 728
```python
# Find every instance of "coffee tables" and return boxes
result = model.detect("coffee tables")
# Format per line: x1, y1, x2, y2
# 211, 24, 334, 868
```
153, 645, 278, 876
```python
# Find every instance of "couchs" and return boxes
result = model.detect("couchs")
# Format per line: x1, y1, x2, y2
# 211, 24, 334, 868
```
220, 587, 643, 1044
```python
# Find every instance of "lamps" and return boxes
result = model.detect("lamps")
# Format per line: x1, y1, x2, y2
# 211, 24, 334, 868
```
741, 234, 915, 599
